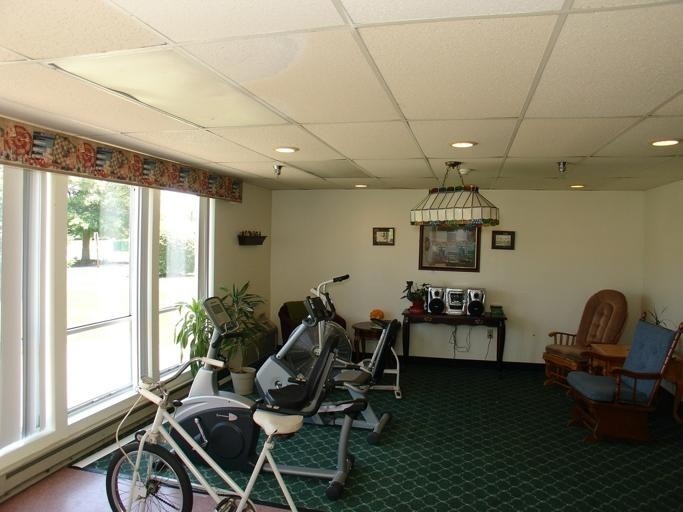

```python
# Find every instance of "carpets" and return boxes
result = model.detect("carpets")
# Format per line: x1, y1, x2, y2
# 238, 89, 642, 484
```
64, 345, 682, 511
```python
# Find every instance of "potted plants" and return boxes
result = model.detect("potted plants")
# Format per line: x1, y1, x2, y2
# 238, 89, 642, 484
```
400, 280, 429, 314
175, 280, 268, 396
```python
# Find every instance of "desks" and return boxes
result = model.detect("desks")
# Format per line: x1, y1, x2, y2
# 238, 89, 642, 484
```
401, 308, 508, 367
352, 321, 399, 366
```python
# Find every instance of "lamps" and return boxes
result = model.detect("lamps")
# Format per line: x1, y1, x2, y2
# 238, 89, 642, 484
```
410, 161, 500, 229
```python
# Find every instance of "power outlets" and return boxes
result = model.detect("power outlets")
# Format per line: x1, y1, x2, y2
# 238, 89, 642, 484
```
487, 328, 493, 339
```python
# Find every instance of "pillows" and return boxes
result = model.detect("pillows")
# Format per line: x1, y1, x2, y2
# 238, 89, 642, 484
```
286, 301, 309, 324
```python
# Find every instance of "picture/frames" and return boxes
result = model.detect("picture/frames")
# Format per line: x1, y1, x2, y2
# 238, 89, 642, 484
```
372, 227, 395, 245
492, 230, 515, 249
418, 225, 479, 272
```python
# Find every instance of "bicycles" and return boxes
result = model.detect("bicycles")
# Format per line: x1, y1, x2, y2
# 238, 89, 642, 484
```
99, 352, 309, 512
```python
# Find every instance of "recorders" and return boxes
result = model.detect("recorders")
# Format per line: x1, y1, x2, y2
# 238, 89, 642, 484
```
427, 286, 486, 317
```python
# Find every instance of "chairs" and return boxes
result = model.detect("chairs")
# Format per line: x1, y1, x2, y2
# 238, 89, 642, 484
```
278, 301, 346, 346
542, 289, 683, 442
